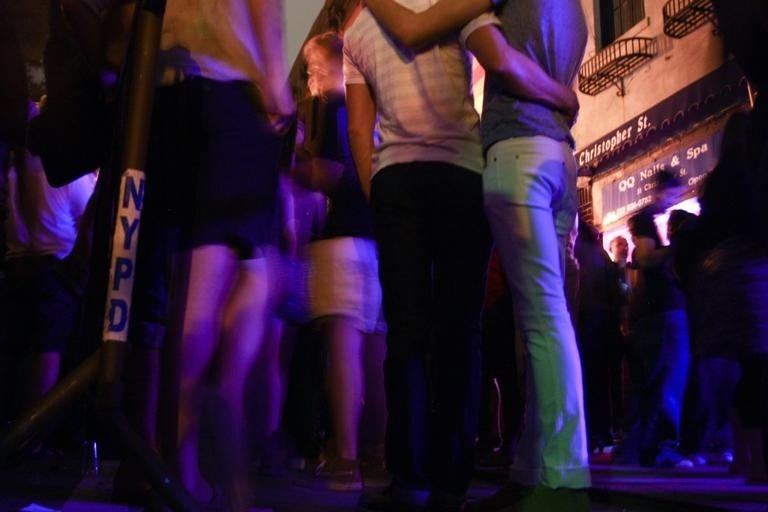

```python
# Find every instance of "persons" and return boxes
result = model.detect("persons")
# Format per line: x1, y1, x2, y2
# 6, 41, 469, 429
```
0, 0, 767, 512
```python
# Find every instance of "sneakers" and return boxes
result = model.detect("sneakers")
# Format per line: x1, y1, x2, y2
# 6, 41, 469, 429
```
465, 481, 593, 511
283, 448, 392, 499
